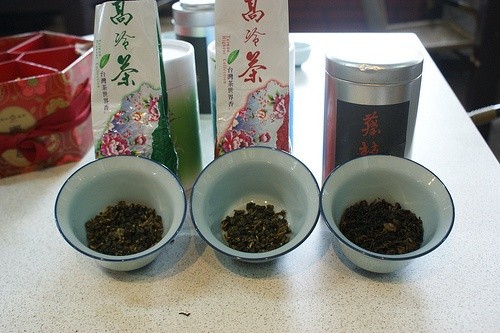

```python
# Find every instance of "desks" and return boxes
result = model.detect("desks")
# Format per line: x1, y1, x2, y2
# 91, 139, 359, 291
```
0, 33, 500, 333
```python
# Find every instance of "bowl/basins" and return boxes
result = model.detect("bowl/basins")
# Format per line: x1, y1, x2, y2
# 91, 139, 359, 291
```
320, 153, 456, 273
190, 145, 321, 263
294, 42, 311, 66
55, 155, 187, 272
